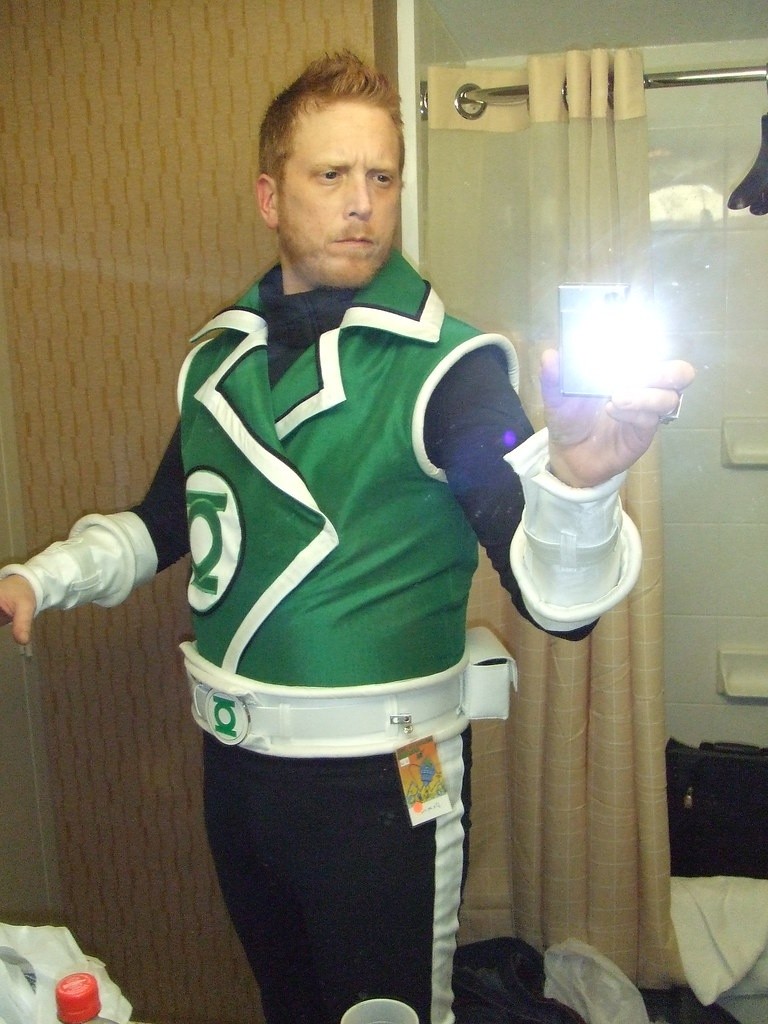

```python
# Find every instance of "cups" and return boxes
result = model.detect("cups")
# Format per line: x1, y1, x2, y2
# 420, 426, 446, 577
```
341, 999, 419, 1024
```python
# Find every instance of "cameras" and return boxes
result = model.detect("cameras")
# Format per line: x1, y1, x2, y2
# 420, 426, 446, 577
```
559, 282, 630, 398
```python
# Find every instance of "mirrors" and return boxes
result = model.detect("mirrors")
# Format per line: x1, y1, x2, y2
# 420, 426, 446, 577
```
0, 0, 768, 1024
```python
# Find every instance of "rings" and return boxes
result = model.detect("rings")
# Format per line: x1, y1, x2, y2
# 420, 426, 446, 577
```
660, 390, 684, 422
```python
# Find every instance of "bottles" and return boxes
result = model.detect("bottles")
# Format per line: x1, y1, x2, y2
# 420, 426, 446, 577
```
56, 974, 119, 1024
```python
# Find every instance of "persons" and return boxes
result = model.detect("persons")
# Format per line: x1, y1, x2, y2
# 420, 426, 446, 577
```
0, 41, 705, 1024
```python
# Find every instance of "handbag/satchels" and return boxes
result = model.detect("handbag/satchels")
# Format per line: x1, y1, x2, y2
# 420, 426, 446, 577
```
663, 738, 768, 878
